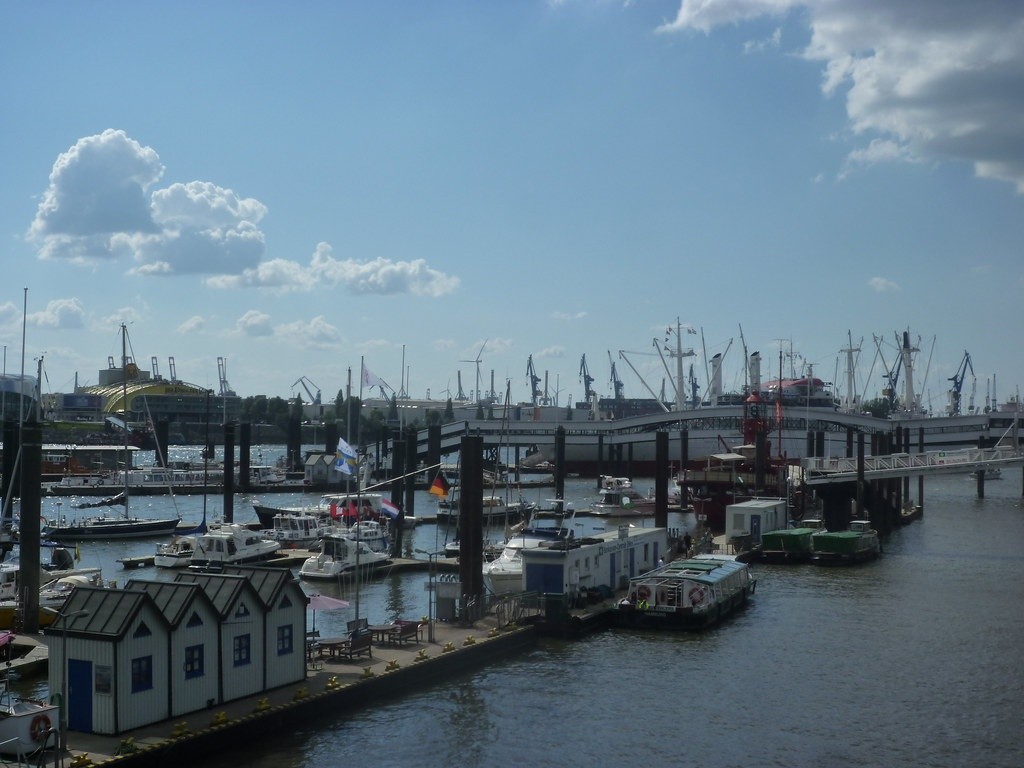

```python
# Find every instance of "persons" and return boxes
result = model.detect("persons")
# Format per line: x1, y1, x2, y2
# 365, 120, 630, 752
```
658, 556, 665, 568
683, 531, 691, 548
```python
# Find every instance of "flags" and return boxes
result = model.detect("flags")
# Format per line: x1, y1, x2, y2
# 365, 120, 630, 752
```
331, 500, 379, 522
334, 437, 357, 476
380, 497, 400, 520
429, 467, 451, 496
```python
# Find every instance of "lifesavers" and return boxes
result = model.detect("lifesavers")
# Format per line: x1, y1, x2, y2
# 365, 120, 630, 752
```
30, 714, 51, 744
688, 588, 704, 603
638, 585, 651, 599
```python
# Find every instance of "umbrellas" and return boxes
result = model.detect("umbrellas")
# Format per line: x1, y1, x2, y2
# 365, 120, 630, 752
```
306, 593, 350, 670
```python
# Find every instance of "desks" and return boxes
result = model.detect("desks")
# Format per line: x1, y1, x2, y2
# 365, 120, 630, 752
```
317, 637, 348, 657
366, 624, 397, 644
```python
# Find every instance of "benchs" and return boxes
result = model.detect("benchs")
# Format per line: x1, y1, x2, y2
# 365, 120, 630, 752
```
306, 631, 322, 655
346, 617, 374, 643
387, 622, 420, 647
340, 635, 373, 661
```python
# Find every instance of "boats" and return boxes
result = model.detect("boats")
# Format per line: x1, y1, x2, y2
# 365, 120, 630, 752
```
0, 429, 1004, 683
